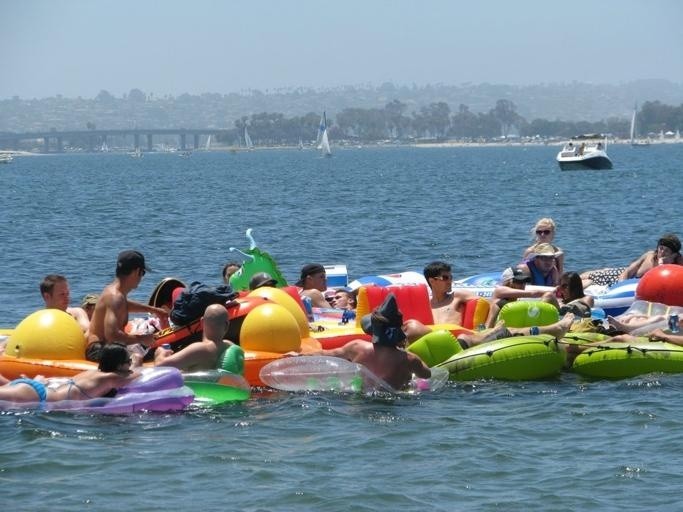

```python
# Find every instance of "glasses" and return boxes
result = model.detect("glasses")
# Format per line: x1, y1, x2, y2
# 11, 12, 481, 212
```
313, 273, 327, 279
140, 270, 146, 276
432, 274, 453, 281
560, 283, 570, 288
534, 229, 553, 235
121, 358, 133, 365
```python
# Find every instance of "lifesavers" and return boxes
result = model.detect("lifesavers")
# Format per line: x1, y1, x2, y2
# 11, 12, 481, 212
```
122, 229, 383, 388
292, 264, 683, 351
409, 329, 569, 382
571, 343, 683, 379
258, 354, 451, 398
0, 308, 102, 380
179, 344, 252, 403
0, 366, 195, 414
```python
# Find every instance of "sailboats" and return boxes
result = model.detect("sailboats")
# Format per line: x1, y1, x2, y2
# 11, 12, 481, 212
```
91, 110, 335, 160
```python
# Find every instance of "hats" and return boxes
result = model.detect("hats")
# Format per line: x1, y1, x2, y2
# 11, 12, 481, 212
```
249, 272, 278, 291
116, 249, 153, 273
293, 263, 326, 287
499, 265, 531, 283
525, 241, 564, 261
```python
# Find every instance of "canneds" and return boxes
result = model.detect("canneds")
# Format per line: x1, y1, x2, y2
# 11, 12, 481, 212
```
161, 343, 171, 357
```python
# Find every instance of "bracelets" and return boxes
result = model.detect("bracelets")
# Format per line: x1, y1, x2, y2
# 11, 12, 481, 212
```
658, 263, 665, 265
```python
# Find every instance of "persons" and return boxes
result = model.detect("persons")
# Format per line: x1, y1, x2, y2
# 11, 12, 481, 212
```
561, 315, 682, 354
578, 143, 586, 155
605, 312, 667, 333
522, 217, 565, 276
541, 270, 595, 318
39, 248, 359, 366
423, 260, 476, 327
0, 340, 143, 406
151, 302, 235, 381
576, 232, 683, 289
597, 143, 604, 152
516, 242, 559, 287
483, 264, 549, 329
400, 310, 574, 350
276, 291, 431, 394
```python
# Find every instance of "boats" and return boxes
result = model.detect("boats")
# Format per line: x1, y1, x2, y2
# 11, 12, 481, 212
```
555, 145, 613, 171
